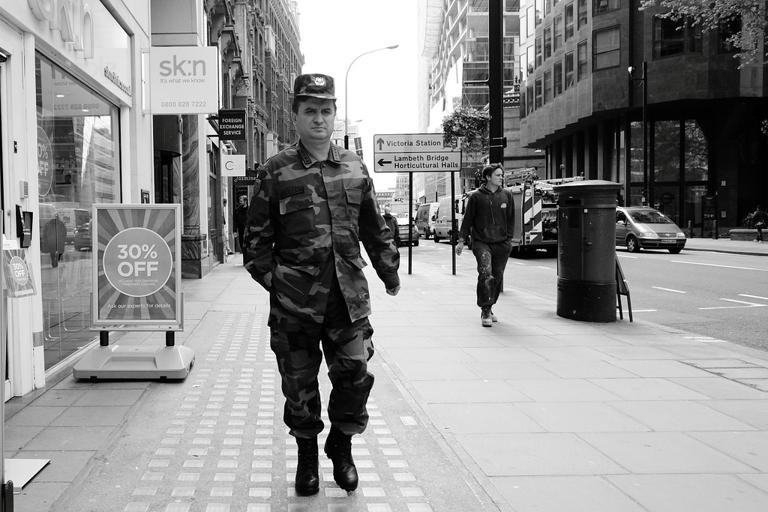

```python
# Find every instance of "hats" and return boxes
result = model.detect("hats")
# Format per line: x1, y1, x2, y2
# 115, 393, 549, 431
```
293, 72, 336, 101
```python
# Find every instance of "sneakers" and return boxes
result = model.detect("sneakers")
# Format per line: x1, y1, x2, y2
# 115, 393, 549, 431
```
481, 307, 499, 328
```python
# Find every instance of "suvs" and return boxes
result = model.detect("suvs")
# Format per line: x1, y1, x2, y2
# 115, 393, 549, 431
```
393, 216, 420, 248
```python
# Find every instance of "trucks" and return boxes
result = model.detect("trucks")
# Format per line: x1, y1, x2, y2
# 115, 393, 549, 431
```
55, 208, 90, 245
384, 195, 467, 243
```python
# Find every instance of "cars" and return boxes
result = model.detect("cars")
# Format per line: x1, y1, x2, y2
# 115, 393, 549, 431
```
616, 203, 687, 255
73, 223, 91, 249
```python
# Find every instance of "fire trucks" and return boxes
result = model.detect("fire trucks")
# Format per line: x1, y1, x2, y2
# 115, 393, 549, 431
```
466, 164, 588, 260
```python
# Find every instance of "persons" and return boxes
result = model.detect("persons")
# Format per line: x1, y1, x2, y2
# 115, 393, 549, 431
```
379, 206, 400, 249
752, 205, 768, 243
455, 164, 514, 327
44, 213, 67, 268
241, 74, 401, 497
232, 195, 250, 250
223, 198, 234, 255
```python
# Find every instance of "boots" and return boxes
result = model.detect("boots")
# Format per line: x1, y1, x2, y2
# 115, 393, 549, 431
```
294, 436, 322, 496
324, 426, 359, 494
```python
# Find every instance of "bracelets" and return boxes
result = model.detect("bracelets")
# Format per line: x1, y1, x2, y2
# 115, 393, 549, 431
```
457, 239, 466, 243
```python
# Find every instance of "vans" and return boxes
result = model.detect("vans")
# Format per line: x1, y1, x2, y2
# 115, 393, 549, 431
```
39, 202, 56, 245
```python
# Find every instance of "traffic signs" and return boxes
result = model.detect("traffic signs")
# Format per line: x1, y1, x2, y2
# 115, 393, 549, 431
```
371, 131, 466, 152
374, 152, 462, 172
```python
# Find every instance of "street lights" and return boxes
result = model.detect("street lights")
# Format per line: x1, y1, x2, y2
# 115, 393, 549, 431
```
332, 118, 364, 155
627, 59, 651, 207
344, 42, 404, 149
